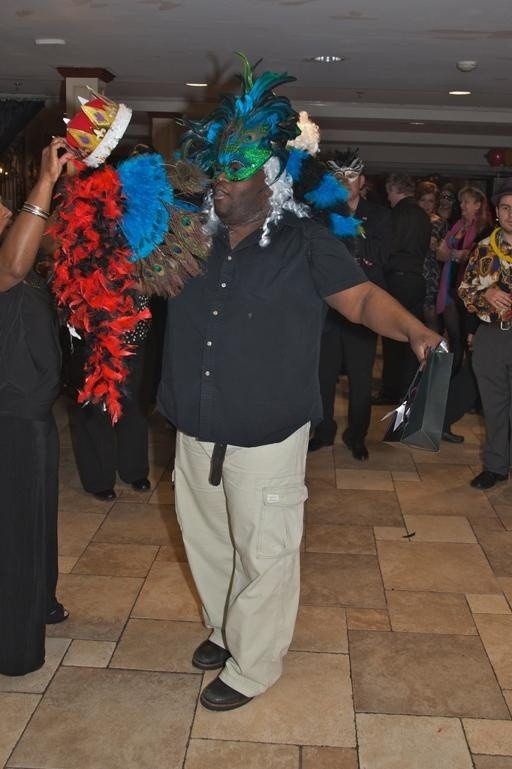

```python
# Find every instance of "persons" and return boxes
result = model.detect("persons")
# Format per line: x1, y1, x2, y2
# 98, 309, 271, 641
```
51, 145, 152, 502
161, 156, 444, 711
458, 181, 512, 490
1, 138, 69, 676
438, 187, 495, 353
374, 171, 432, 404
415, 180, 446, 329
307, 151, 391, 461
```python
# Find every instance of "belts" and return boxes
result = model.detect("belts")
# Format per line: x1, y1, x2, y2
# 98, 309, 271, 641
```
208, 442, 227, 486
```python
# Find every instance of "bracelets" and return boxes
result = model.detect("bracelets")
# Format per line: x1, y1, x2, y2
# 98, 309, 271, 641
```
23, 201, 52, 223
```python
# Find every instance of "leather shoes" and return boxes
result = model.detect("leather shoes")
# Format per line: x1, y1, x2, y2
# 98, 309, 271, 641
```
307, 435, 335, 453
192, 641, 232, 669
469, 469, 508, 490
133, 476, 151, 492
200, 673, 257, 711
96, 486, 116, 501
341, 432, 369, 460
372, 390, 401, 405
441, 428, 464, 445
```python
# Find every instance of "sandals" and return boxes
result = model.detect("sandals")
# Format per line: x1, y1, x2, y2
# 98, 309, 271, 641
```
46, 601, 69, 623
